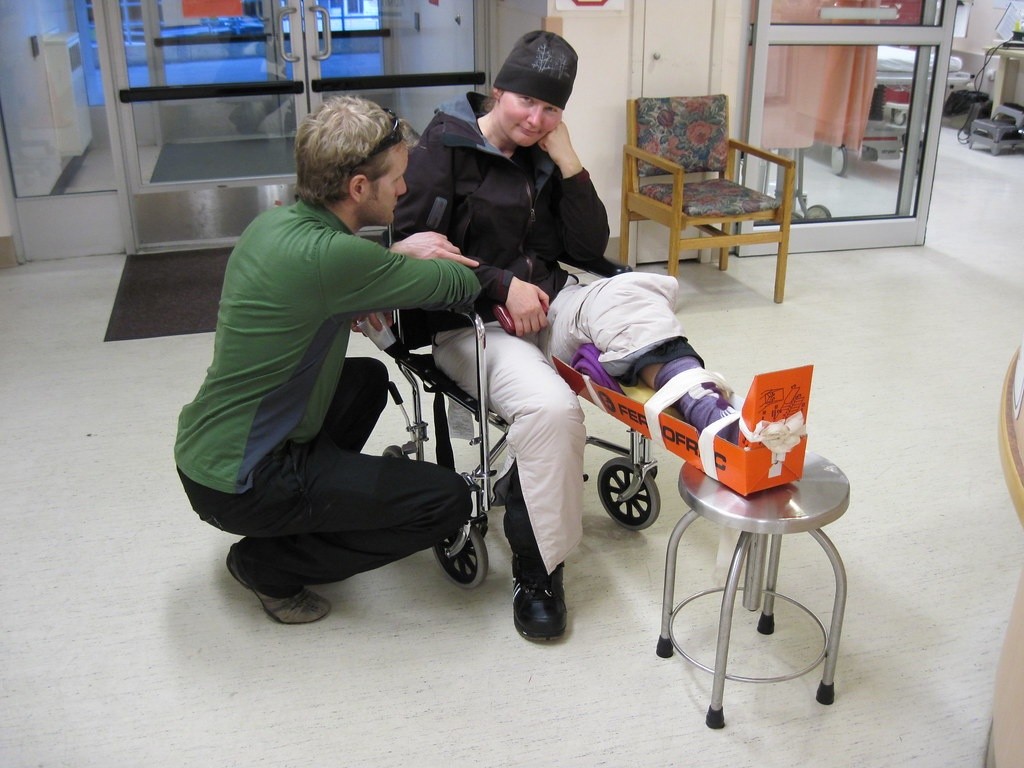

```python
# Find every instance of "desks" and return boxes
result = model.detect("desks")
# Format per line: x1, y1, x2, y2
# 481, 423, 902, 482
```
983, 46, 1024, 120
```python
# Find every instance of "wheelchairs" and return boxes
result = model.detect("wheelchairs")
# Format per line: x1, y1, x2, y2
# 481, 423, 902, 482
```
371, 228, 663, 586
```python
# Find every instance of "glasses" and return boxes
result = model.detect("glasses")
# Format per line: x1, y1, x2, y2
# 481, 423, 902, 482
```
349, 107, 403, 176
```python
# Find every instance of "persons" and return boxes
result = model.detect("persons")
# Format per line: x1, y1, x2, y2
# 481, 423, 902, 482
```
380, 30, 813, 640
173, 96, 482, 625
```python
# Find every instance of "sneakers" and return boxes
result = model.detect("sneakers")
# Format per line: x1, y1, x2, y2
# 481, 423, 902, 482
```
225, 543, 332, 625
510, 555, 568, 642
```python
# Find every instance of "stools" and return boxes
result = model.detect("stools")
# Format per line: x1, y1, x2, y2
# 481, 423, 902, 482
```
656, 452, 850, 729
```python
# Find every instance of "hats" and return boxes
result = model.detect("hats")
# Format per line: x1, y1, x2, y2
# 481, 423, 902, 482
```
493, 30, 579, 111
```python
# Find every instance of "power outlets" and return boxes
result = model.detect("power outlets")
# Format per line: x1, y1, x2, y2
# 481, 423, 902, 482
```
988, 69, 996, 81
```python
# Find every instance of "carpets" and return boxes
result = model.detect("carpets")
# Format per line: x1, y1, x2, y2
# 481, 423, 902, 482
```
149, 137, 296, 183
102, 235, 387, 341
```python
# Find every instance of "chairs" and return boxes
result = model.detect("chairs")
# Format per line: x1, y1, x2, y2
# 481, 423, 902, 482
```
619, 92, 795, 305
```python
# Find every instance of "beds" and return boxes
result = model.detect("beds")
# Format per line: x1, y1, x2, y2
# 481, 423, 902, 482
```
831, 46, 969, 176
